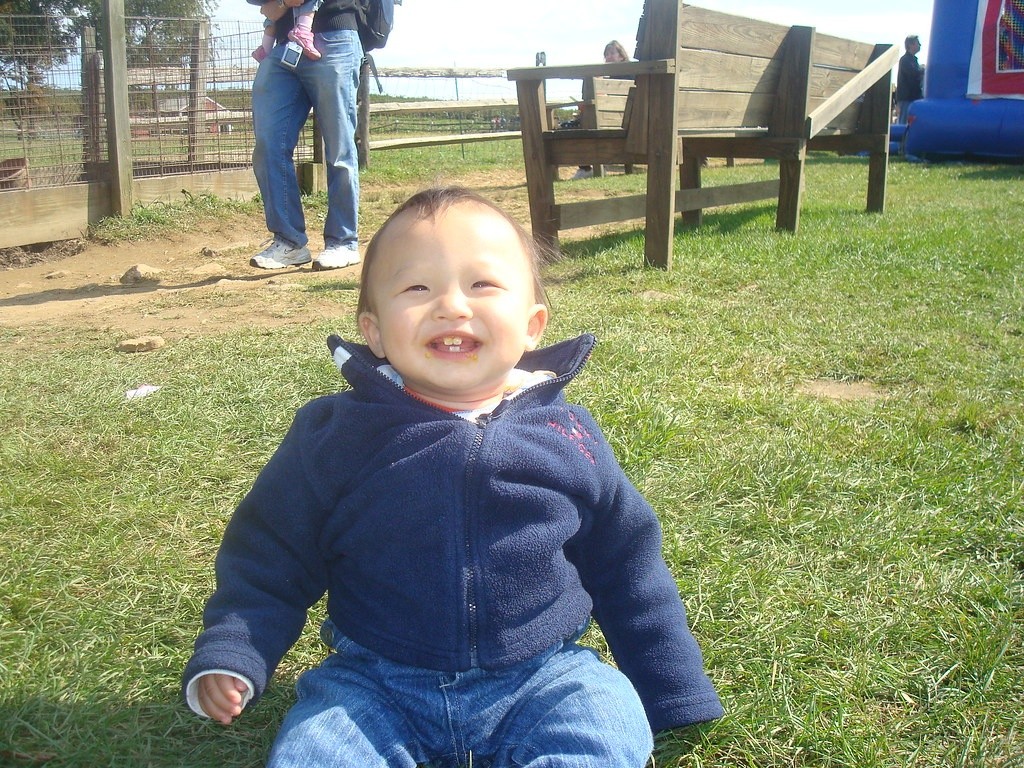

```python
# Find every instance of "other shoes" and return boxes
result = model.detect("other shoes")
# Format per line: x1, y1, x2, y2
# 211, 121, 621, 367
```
287, 26, 321, 60
251, 45, 267, 63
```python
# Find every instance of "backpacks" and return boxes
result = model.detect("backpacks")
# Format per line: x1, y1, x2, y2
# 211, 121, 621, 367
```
355, 0, 402, 94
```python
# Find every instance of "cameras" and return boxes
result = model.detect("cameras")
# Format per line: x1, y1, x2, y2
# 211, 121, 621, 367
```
280, 41, 303, 69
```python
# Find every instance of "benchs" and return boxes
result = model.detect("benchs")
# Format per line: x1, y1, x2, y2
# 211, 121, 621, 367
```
506, 0, 900, 271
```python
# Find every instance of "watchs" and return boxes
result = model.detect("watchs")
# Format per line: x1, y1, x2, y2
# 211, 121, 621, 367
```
277, 0, 287, 9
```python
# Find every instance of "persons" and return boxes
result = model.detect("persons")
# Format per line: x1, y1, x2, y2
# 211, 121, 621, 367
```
178, 186, 728, 768
896, 35, 925, 125
560, 40, 637, 180
490, 113, 506, 130
246, 0, 370, 269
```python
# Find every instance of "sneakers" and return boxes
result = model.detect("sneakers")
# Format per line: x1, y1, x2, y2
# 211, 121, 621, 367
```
312, 242, 360, 270
573, 168, 592, 180
250, 238, 311, 268
